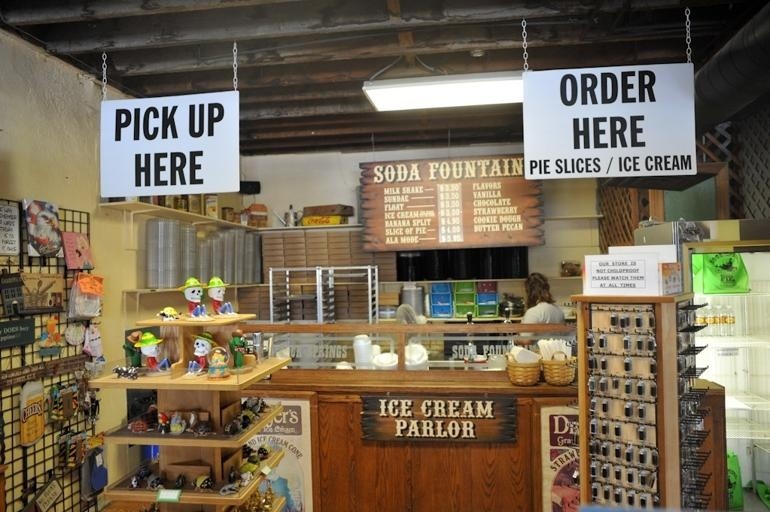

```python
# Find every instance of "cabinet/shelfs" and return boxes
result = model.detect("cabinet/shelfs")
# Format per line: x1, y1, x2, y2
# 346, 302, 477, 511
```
571, 291, 713, 510
99, 200, 609, 331
88, 310, 293, 511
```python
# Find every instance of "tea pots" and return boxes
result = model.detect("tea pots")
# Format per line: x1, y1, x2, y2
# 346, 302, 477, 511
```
500, 295, 524, 318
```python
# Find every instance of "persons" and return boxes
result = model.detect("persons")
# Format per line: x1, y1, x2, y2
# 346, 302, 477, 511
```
515, 271, 568, 350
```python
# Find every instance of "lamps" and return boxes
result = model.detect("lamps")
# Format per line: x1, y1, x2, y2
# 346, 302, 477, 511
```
358, 54, 541, 114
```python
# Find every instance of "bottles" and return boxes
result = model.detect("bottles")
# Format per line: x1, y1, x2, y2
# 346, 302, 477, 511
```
693, 304, 738, 336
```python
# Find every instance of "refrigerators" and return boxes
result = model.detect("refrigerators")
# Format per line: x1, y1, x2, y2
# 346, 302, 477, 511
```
630, 212, 770, 511
